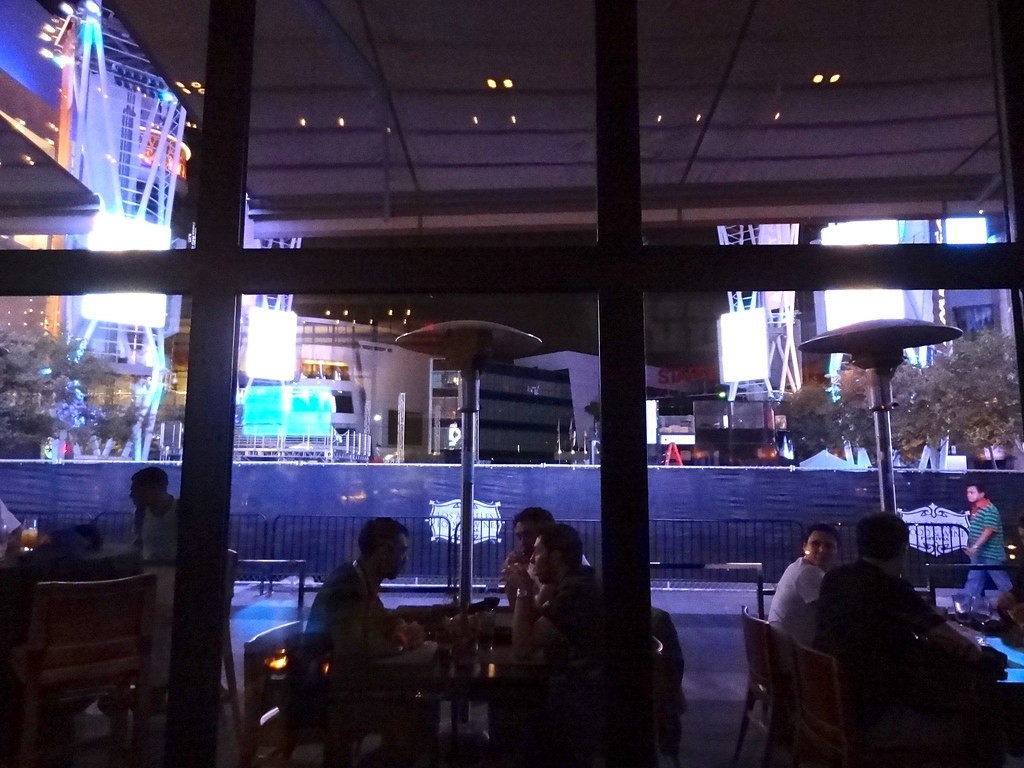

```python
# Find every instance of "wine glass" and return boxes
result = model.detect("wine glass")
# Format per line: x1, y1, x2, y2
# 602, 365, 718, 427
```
972, 600, 993, 646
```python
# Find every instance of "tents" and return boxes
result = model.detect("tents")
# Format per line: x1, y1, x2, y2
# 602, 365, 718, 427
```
800, 451, 865, 468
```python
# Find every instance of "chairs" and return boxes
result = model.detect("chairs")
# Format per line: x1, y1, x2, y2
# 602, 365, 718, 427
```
11, 572, 161, 767
733, 604, 794, 768
789, 638, 908, 768
162, 547, 239, 736
241, 619, 333, 768
567, 635, 663, 768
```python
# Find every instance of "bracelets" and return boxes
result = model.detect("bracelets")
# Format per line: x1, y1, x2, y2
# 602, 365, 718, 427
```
516, 591, 529, 599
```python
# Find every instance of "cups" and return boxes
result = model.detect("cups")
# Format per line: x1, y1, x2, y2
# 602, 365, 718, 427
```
20, 519, 38, 551
475, 611, 496, 649
952, 594, 973, 622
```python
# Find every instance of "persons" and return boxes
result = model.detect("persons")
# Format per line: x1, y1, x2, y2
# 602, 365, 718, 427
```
574, 595, 685, 768
812, 512, 984, 768
965, 484, 1012, 597
487, 525, 602, 756
767, 525, 840, 708
501, 507, 591, 607
0, 467, 181, 749
999, 515, 1024, 628
286, 517, 441, 768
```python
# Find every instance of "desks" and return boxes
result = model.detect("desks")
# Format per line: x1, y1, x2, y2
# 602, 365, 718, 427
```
328, 605, 549, 768
940, 620, 1023, 768
0, 540, 131, 573
